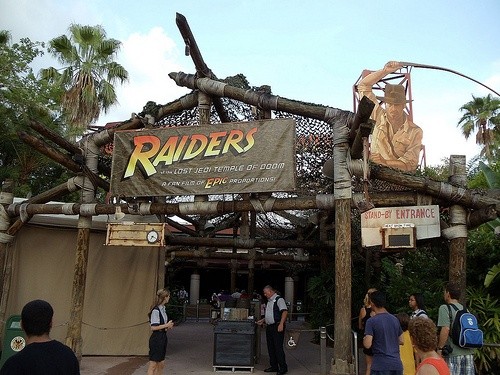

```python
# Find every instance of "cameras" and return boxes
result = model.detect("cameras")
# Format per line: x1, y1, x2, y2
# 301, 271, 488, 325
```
442, 345, 453, 356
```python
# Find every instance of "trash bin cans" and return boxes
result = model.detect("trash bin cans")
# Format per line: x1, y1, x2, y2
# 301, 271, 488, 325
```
1, 315, 27, 364
284, 329, 300, 350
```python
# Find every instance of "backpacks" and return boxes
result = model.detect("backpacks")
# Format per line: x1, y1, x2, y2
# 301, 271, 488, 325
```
447, 302, 484, 348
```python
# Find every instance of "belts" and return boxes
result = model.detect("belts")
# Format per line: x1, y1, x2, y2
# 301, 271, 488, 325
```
266, 321, 280, 327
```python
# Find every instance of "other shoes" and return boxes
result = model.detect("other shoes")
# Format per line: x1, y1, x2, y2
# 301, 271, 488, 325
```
264, 365, 279, 372
277, 367, 288, 374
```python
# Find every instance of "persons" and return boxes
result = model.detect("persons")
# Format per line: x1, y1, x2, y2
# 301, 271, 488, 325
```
358, 61, 423, 170
408, 294, 428, 318
358, 288, 377, 375
212, 289, 252, 307
409, 316, 450, 375
177, 286, 188, 303
147, 287, 174, 375
0, 299, 81, 375
364, 290, 404, 375
438, 282, 478, 375
396, 313, 415, 375
257, 285, 289, 375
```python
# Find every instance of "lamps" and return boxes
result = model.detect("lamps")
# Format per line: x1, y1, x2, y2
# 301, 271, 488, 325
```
204, 223, 215, 232
114, 193, 138, 220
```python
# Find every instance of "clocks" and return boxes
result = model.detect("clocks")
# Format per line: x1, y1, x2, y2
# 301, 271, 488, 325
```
146, 230, 158, 244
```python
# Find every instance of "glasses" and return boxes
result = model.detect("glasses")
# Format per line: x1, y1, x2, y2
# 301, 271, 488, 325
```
409, 299, 416, 302
